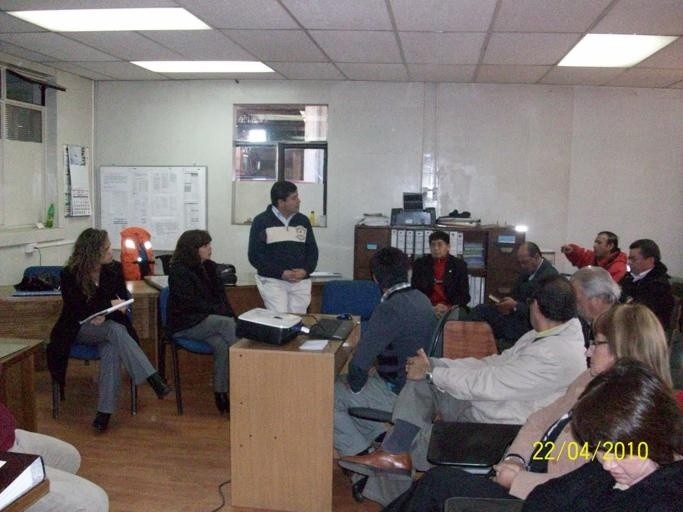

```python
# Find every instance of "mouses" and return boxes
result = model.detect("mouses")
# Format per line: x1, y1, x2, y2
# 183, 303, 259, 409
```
336, 312, 352, 320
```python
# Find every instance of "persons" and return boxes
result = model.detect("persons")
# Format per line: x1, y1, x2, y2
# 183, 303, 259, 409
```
47, 227, 171, 435
0, 399, 107, 508
334, 230, 682, 511
155, 228, 246, 418
245, 180, 320, 315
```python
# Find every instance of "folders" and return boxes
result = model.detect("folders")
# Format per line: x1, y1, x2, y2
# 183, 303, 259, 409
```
390, 229, 464, 258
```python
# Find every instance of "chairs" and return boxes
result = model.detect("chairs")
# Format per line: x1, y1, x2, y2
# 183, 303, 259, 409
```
159, 285, 216, 417
662, 295, 683, 357
427, 422, 523, 468
347, 304, 460, 503
320, 279, 382, 321
50, 289, 140, 420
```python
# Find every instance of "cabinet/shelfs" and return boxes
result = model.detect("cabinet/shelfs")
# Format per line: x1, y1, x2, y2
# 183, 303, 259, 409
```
353, 219, 527, 307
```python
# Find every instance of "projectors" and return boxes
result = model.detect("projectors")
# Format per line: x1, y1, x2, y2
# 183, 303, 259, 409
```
236, 306, 303, 346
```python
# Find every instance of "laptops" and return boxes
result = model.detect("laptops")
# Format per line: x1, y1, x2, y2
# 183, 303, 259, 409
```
309, 317, 354, 341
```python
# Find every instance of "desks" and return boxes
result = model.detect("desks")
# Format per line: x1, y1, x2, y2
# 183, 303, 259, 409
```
0, 278, 162, 388
228, 312, 362, 512
0, 336, 45, 433
143, 271, 350, 388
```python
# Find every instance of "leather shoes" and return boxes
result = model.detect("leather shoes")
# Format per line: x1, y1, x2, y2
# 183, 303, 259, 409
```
214, 391, 230, 417
337, 447, 413, 482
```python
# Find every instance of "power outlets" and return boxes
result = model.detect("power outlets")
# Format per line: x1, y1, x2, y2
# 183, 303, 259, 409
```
24, 242, 37, 253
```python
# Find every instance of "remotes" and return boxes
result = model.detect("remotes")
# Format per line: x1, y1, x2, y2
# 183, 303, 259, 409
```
561, 246, 566, 253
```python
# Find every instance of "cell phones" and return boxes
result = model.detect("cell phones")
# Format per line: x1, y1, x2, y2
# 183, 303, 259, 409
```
488, 292, 502, 304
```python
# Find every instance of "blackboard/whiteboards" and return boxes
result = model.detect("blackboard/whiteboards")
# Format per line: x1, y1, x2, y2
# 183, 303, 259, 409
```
98, 165, 208, 252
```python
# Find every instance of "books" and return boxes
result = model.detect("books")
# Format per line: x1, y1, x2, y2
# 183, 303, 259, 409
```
0, 449, 45, 511
78, 298, 136, 322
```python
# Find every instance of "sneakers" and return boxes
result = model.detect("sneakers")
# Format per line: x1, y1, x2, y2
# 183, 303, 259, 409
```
146, 371, 173, 400
91, 410, 112, 433
